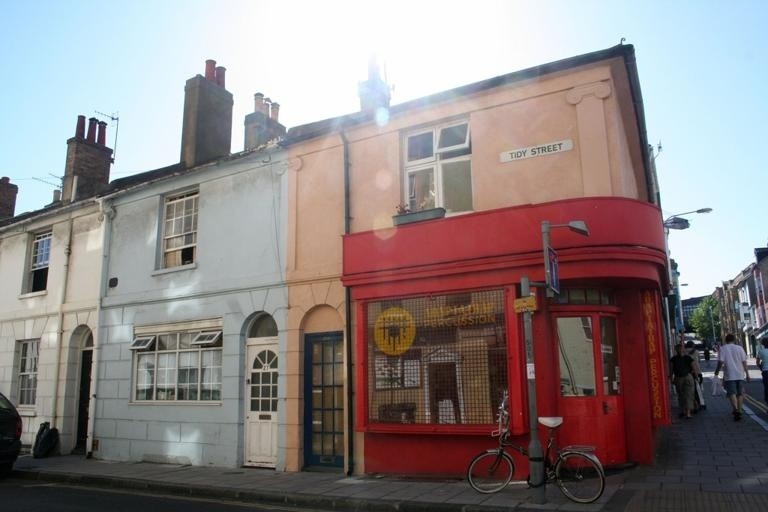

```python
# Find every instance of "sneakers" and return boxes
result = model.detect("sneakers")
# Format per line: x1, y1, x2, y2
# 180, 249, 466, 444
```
734, 408, 746, 418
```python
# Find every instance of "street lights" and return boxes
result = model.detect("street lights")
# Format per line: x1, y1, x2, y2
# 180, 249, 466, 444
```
519, 220, 590, 503
663, 208, 713, 291
709, 306, 716, 342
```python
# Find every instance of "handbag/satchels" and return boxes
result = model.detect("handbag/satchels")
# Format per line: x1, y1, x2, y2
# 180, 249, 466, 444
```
710, 375, 722, 397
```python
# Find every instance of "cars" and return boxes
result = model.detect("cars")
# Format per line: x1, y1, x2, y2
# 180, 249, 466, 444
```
0, 392, 23, 475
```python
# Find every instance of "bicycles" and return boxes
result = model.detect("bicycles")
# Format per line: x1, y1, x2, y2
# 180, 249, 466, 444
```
467, 395, 605, 505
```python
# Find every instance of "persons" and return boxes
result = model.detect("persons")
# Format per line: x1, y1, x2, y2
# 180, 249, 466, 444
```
755, 337, 768, 405
686, 341, 702, 414
669, 344, 698, 420
704, 347, 711, 362
715, 333, 751, 421
